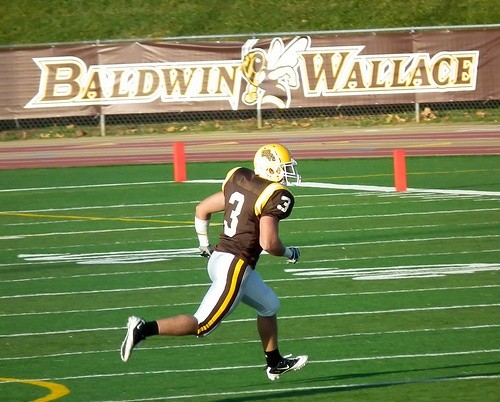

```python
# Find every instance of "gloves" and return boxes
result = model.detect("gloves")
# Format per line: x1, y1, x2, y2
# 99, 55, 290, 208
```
287, 247, 301, 264
199, 244, 213, 258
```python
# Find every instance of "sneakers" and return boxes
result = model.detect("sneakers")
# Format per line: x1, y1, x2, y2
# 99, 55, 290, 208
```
266, 354, 308, 380
119, 316, 144, 362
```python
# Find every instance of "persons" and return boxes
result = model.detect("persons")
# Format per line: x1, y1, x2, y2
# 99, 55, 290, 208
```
121, 144, 308, 380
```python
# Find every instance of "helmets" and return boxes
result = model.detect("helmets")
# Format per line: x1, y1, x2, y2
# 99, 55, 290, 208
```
253, 143, 293, 182
